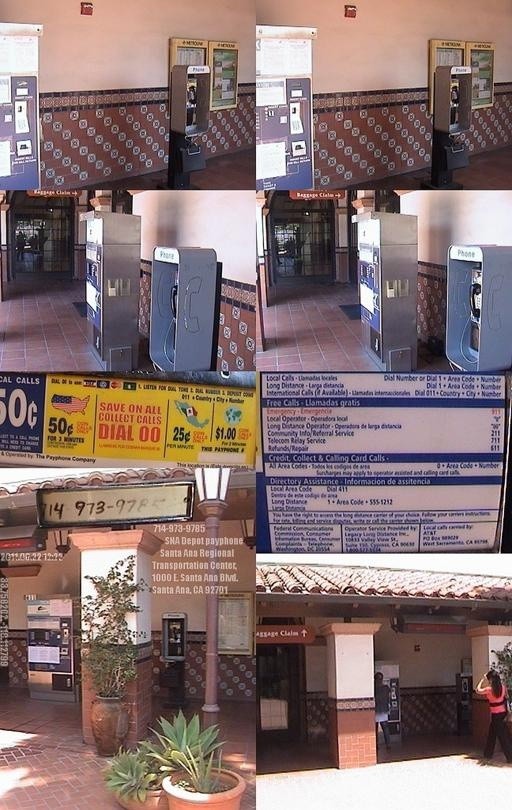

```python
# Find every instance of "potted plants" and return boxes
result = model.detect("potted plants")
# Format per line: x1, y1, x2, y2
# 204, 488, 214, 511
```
100, 705, 247, 810
490, 642, 512, 753
61, 553, 154, 757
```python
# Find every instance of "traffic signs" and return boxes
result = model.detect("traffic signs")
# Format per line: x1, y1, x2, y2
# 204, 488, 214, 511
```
256, 624, 316, 645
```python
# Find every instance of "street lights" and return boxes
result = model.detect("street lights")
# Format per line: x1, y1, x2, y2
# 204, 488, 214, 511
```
194, 466, 232, 759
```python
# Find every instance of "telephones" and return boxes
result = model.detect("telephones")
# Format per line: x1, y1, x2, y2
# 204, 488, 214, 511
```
450, 79, 461, 108
186, 77, 198, 110
170, 286, 178, 319
469, 267, 482, 322
169, 621, 183, 645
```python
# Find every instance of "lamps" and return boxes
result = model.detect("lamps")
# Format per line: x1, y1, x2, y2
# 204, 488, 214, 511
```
54, 528, 72, 556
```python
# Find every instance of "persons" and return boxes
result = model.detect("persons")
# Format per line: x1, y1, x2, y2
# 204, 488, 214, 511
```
16, 230, 38, 260
374, 671, 391, 750
275, 236, 296, 264
473, 669, 512, 763
